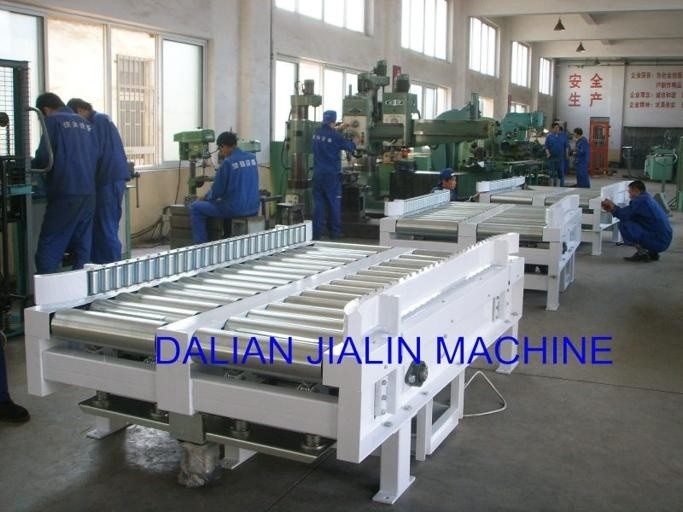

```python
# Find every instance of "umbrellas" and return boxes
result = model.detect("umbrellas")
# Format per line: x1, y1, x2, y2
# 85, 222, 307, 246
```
624, 252, 659, 262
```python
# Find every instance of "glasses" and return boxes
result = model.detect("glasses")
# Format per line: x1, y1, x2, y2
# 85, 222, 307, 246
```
552, 18, 590, 57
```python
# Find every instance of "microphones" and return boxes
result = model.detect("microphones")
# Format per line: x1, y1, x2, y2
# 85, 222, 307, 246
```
275, 201, 305, 225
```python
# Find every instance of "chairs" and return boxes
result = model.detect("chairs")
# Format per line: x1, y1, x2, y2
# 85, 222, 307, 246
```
1, 399, 30, 422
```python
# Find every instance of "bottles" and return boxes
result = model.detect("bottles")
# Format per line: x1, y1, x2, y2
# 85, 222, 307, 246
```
321, 110, 336, 126
440, 168, 455, 177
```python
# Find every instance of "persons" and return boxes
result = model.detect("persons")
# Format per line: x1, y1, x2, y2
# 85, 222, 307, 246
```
601, 180, 673, 261
312, 110, 361, 240
31, 93, 100, 274
544, 123, 568, 187
67, 98, 131, 264
571, 128, 591, 188
431, 168, 480, 202
0, 337, 31, 422
189, 131, 261, 245
